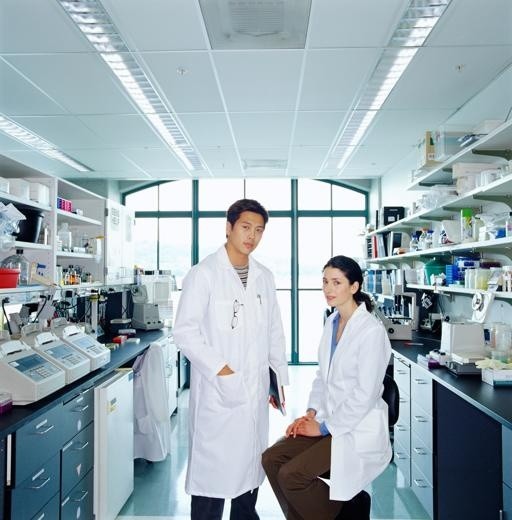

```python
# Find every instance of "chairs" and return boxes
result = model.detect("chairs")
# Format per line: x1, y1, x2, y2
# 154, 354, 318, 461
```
381, 372, 399, 424
133, 338, 169, 467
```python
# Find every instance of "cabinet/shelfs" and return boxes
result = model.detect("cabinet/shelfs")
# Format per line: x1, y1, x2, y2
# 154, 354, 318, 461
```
0, 155, 138, 294
355, 116, 511, 304
392, 358, 506, 520
175, 349, 193, 396
11, 374, 132, 520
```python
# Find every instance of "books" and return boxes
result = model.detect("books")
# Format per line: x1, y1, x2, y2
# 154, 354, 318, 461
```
267, 360, 287, 416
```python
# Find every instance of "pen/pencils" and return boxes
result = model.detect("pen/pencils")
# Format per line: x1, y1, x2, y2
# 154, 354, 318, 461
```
404, 342, 424, 346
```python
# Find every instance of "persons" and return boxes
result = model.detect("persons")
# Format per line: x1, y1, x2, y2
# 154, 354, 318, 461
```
260, 255, 395, 519
172, 198, 291, 520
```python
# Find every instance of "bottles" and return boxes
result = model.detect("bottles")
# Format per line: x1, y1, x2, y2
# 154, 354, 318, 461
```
65, 288, 118, 333
405, 159, 512, 368
1, 175, 103, 287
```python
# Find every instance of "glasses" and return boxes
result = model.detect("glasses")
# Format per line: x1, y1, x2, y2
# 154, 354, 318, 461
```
230, 300, 241, 329
